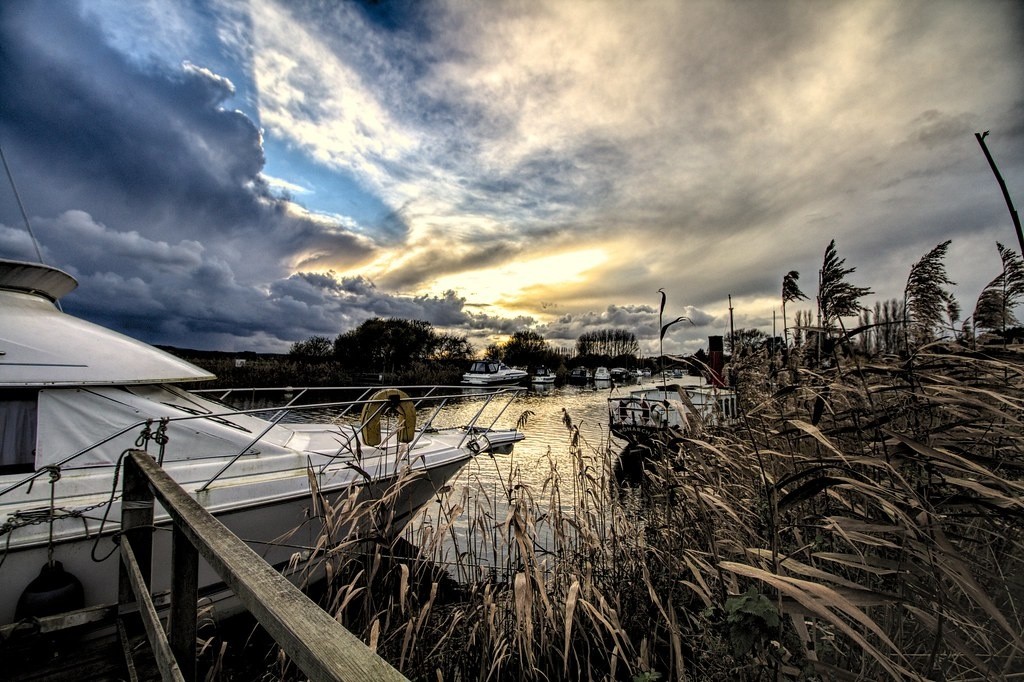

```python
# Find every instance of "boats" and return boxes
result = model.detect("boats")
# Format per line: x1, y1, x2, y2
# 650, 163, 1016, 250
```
571, 366, 592, 380
594, 366, 611, 379
611, 368, 629, 380
461, 359, 528, 384
531, 365, 556, 383
0, 148, 526, 682
607, 384, 736, 448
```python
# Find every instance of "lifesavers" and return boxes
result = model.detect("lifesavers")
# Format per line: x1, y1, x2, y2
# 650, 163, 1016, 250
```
360, 387, 418, 447
617, 395, 650, 426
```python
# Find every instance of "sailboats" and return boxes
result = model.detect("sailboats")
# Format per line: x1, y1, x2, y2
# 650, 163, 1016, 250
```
630, 349, 651, 377
660, 352, 683, 379
